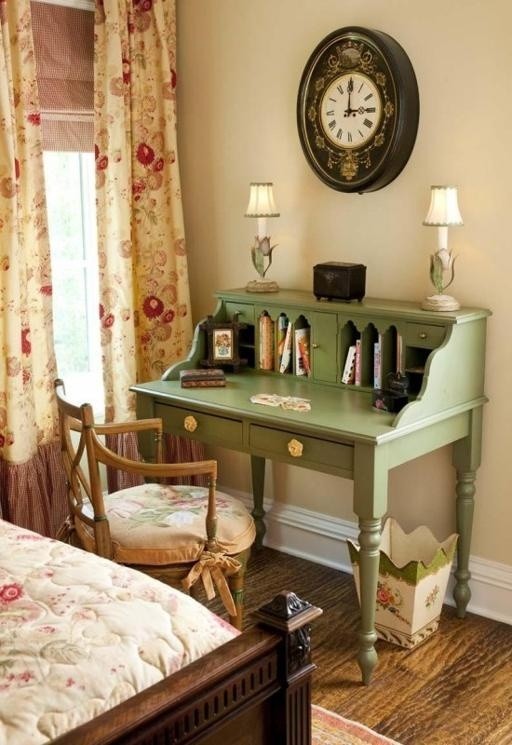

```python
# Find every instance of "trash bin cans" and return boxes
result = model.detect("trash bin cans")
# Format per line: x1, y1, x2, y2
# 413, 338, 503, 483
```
346, 517, 460, 650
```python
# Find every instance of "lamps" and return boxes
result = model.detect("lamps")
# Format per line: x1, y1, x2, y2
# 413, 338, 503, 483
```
245, 182, 280, 292
422, 185, 464, 312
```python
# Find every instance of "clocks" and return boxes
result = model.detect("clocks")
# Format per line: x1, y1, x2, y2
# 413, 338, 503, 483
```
297, 27, 419, 193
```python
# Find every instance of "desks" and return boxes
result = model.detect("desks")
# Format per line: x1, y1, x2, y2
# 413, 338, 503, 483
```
129, 285, 494, 683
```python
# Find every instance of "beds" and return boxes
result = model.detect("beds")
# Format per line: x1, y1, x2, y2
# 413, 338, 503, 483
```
0, 518, 324, 745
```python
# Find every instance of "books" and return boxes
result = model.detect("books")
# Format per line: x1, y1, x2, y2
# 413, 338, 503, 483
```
258, 316, 402, 390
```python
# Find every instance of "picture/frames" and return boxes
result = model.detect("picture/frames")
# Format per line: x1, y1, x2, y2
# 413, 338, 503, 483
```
200, 313, 247, 373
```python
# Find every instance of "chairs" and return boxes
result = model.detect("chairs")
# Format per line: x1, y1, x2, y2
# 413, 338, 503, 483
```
53, 377, 256, 631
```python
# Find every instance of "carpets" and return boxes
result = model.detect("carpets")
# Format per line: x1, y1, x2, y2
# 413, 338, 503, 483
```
310, 704, 401, 745
